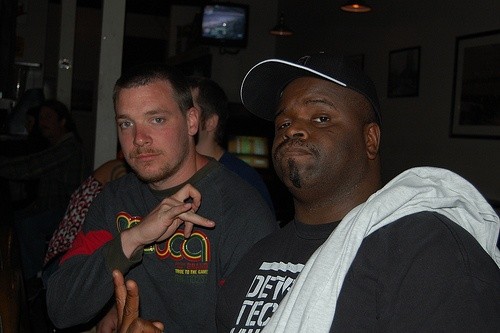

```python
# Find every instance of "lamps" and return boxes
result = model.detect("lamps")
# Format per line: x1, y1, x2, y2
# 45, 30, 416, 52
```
267, 0, 295, 36
336, 0, 372, 14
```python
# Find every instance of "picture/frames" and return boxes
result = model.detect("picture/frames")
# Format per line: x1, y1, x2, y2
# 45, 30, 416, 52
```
336, 54, 364, 71
386, 46, 422, 98
449, 30, 500, 140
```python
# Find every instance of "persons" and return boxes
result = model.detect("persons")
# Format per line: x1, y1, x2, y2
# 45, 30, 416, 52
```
113, 52, 500, 333
0, 99, 85, 302
44, 56, 278, 333
45, 146, 129, 267
190, 76, 275, 216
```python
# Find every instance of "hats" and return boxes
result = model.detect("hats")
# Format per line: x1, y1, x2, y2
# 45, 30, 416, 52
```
240, 50, 383, 129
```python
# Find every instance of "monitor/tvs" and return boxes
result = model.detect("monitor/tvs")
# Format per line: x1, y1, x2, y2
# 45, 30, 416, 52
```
191, 1, 249, 49
